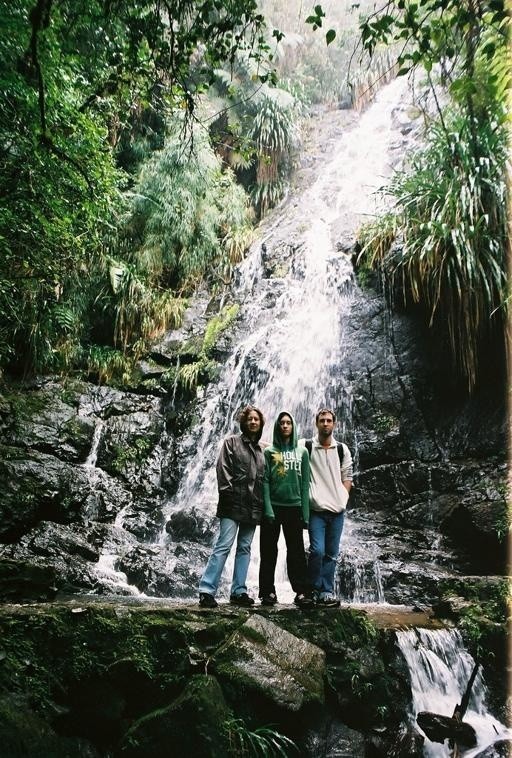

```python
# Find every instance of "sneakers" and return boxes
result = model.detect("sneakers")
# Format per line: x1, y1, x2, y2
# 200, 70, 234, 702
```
229, 593, 255, 604
311, 589, 320, 604
315, 599, 340, 607
199, 592, 218, 608
293, 594, 313, 607
260, 594, 276, 606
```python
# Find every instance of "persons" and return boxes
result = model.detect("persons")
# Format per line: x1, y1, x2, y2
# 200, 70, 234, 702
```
197, 405, 265, 607
256, 410, 311, 605
296, 407, 353, 607
